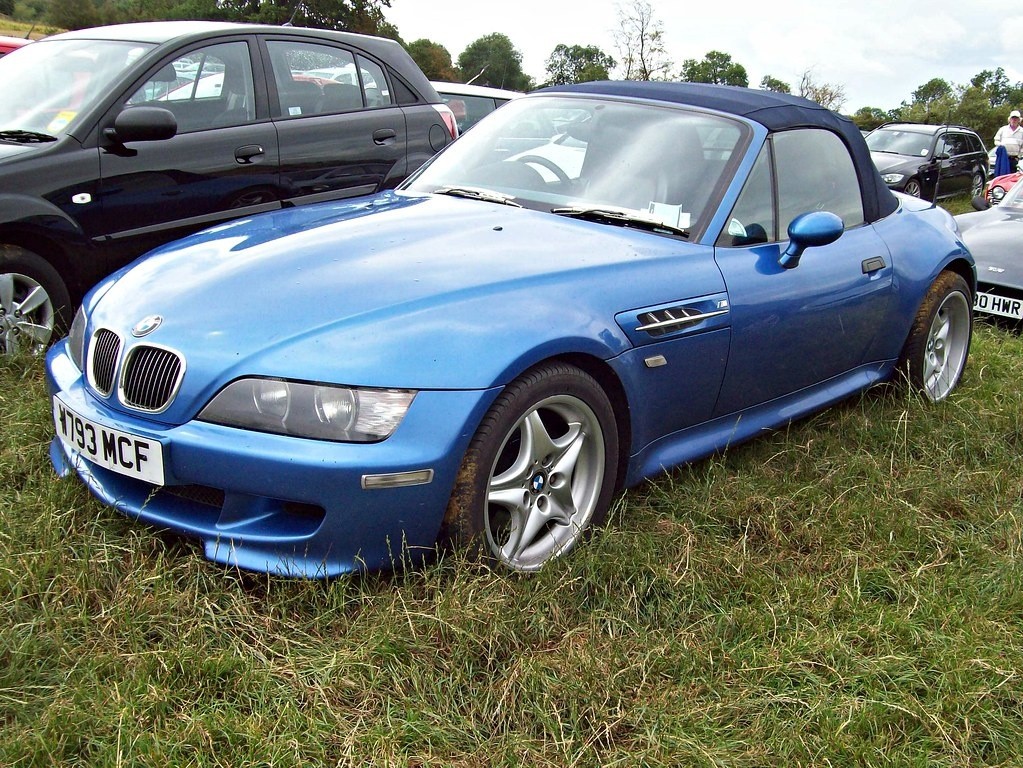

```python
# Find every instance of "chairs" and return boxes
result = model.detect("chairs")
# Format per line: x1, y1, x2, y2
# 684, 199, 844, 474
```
734, 135, 839, 227
136, 65, 176, 123
598, 119, 706, 204
211, 106, 247, 128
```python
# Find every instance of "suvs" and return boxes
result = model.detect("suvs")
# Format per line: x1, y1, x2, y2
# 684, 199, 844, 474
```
858, 121, 990, 203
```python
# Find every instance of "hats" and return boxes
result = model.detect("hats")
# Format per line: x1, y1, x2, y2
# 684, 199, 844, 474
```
1010, 110, 1020, 118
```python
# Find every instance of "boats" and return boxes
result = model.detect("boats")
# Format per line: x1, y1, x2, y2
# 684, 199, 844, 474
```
0, 24, 459, 363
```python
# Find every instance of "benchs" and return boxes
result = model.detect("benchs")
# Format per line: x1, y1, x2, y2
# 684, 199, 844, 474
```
283, 82, 362, 116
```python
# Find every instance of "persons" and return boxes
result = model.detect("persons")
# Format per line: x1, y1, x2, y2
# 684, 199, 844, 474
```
994, 111, 1023, 179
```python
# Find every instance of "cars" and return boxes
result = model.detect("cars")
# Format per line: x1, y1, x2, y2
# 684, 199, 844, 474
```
0, 36, 740, 183
45, 80, 977, 577
951, 146, 1023, 319
